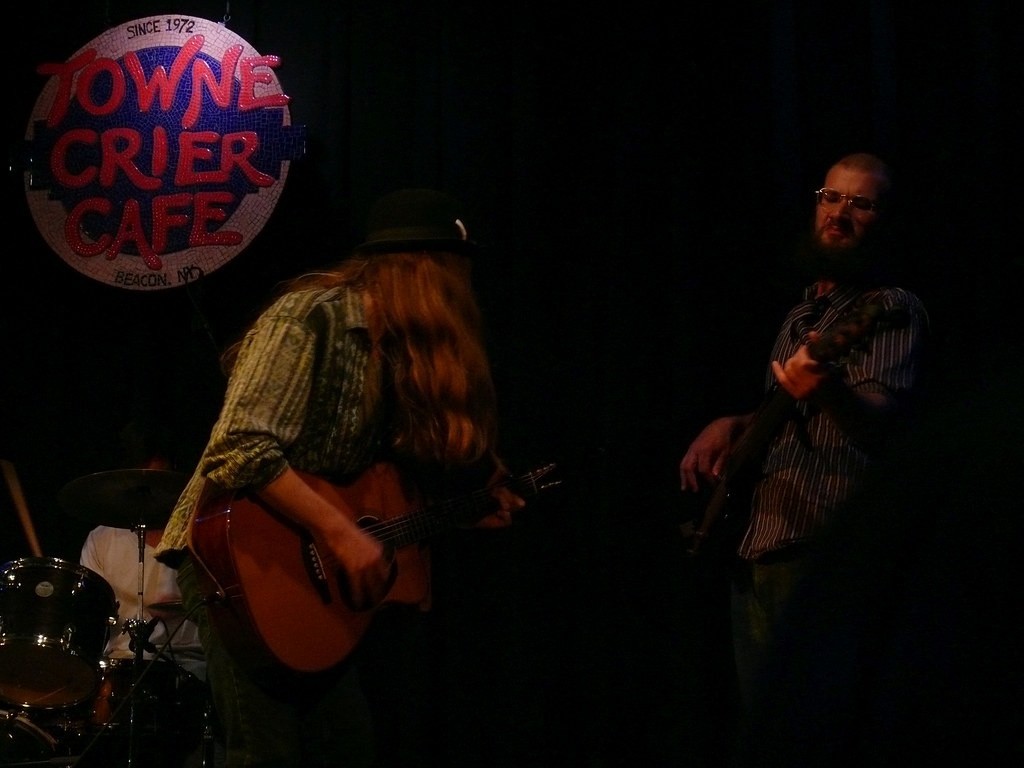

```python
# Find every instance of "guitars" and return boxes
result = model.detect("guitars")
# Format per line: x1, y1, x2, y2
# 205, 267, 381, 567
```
671, 296, 897, 556
195, 459, 563, 682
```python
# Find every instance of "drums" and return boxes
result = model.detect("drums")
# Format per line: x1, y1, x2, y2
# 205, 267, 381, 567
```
0, 556, 120, 713
88, 658, 212, 768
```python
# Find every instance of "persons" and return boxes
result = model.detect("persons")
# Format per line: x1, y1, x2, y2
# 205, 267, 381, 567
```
151, 245, 526, 678
78, 501, 203, 662
679, 154, 922, 576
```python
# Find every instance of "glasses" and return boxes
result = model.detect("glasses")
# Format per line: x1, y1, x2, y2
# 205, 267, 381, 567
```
814, 187, 883, 216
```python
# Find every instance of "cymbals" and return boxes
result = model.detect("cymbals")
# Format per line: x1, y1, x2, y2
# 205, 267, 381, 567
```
55, 469, 190, 530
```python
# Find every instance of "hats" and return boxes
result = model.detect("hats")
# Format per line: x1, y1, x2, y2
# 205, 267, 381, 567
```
342, 185, 465, 248
